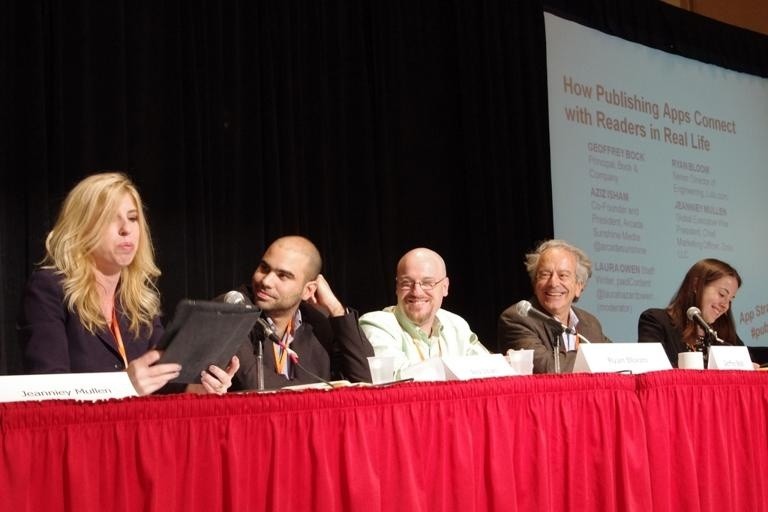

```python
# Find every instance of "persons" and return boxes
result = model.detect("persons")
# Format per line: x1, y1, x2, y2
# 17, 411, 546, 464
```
21, 173, 241, 397
357, 247, 492, 385
500, 238, 615, 374
636, 257, 766, 369
230, 235, 373, 391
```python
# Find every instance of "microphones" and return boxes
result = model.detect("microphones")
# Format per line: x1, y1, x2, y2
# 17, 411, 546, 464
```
516, 299, 574, 335
687, 306, 724, 346
224, 290, 279, 342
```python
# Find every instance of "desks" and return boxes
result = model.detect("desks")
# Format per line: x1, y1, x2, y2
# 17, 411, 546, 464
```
1, 367, 768, 512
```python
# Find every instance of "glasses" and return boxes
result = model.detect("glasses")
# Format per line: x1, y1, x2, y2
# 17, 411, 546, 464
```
398, 277, 446, 291
